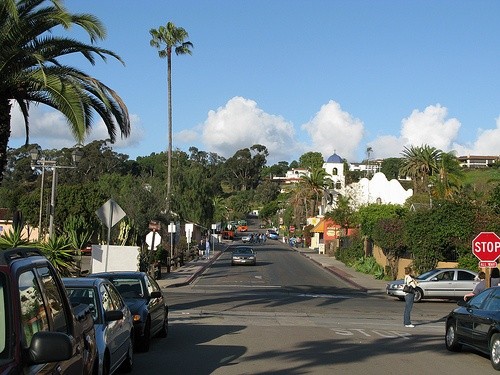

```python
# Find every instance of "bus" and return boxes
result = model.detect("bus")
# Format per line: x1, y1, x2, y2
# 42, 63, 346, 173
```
238, 222, 248, 232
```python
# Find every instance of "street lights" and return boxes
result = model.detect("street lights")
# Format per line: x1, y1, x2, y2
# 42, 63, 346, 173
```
30, 145, 85, 243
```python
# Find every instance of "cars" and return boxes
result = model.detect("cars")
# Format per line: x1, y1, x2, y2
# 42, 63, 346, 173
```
62, 277, 134, 375
69, 271, 168, 352
445, 285, 500, 371
232, 246, 256, 265
265, 229, 279, 240
242, 233, 257, 243
385, 267, 481, 302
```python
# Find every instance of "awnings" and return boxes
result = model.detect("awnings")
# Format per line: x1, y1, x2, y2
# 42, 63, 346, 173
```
310, 220, 324, 233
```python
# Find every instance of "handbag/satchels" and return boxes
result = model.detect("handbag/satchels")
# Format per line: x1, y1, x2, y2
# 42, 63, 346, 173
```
403, 277, 414, 293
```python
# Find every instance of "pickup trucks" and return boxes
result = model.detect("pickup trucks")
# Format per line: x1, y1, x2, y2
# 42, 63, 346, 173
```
0, 246, 96, 375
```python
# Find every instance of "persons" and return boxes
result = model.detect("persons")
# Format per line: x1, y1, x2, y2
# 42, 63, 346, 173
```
443, 273, 451, 281
199, 238, 211, 260
404, 267, 417, 328
251, 233, 267, 244
290, 235, 304, 248
464, 272, 486, 301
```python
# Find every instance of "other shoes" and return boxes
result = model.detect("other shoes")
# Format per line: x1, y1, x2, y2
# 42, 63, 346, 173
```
404, 324, 414, 328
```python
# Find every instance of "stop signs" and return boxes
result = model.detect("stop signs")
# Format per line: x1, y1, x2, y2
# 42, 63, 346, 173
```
472, 232, 500, 262
